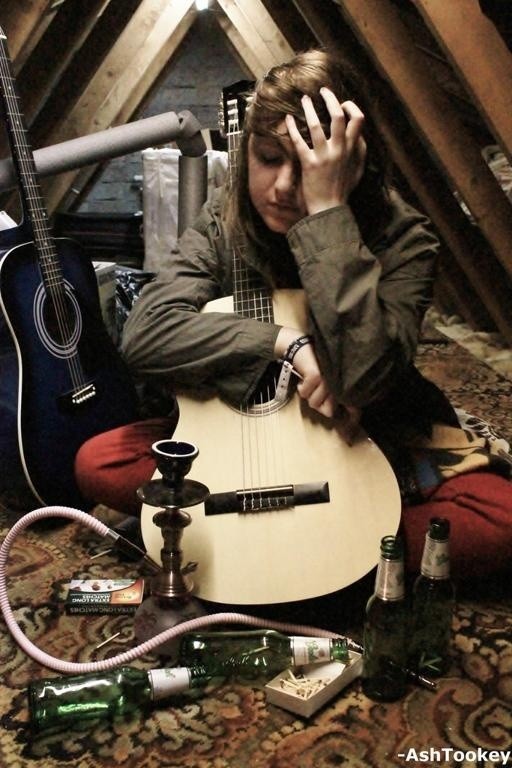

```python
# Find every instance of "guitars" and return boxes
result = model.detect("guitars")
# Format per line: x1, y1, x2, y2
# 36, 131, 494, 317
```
1, 27, 147, 526
140, 79, 402, 627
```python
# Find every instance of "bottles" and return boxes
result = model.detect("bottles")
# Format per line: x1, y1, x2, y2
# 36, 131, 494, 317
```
29, 666, 207, 730
363, 535, 410, 703
180, 630, 348, 679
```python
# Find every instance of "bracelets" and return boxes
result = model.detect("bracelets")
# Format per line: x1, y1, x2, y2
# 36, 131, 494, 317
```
282, 336, 311, 368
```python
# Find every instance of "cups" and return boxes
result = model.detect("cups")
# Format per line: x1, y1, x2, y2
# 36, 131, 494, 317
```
410, 518, 455, 678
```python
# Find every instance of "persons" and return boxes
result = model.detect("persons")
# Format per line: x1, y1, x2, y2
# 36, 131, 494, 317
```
75, 50, 510, 573
